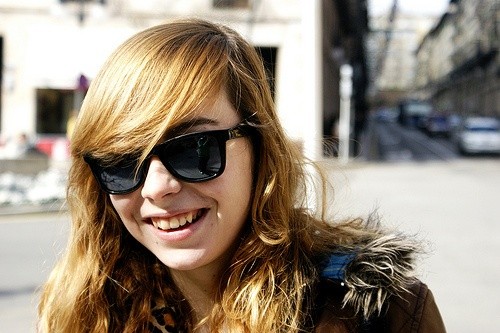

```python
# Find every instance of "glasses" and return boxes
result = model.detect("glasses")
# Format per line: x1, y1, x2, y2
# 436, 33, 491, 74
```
82, 125, 249, 195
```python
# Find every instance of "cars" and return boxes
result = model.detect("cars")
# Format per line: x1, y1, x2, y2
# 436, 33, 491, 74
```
400, 97, 454, 137
455, 115, 500, 155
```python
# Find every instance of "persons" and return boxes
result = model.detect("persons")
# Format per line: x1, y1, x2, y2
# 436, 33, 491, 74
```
35, 17, 451, 333
